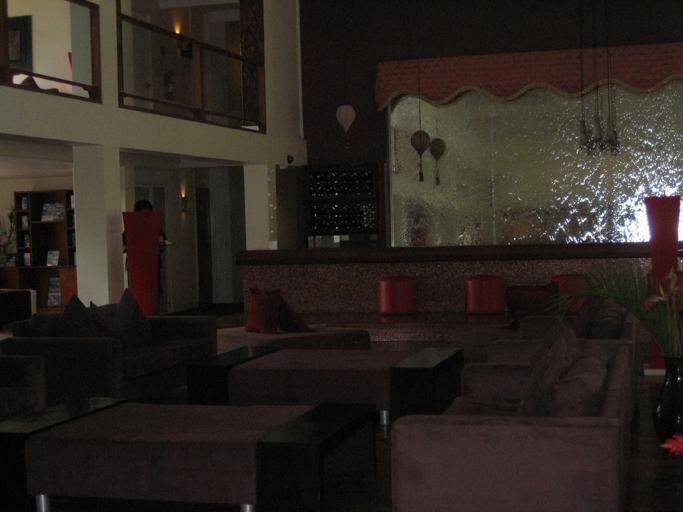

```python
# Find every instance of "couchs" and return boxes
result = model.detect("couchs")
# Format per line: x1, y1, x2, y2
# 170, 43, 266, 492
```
0, 310, 218, 408
217, 247, 682, 363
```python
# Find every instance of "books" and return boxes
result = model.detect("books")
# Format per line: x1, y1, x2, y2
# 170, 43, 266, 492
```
18, 196, 76, 306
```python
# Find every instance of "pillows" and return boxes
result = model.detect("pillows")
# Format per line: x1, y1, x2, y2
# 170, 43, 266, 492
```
28, 288, 155, 347
571, 295, 624, 337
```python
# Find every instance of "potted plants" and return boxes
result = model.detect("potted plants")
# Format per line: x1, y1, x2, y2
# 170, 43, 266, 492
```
582, 258, 683, 458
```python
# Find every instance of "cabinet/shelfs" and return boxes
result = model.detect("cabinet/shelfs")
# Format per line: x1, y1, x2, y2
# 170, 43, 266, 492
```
0, 189, 75, 308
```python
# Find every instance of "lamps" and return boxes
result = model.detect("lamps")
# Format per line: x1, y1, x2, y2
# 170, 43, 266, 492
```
410, 78, 430, 182
333, 0, 359, 139
429, 104, 448, 187
574, 0, 620, 154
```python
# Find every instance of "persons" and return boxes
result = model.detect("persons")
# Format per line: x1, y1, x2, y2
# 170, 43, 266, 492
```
122, 197, 173, 304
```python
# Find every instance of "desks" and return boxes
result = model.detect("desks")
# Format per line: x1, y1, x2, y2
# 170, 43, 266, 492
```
0, 346, 463, 510
